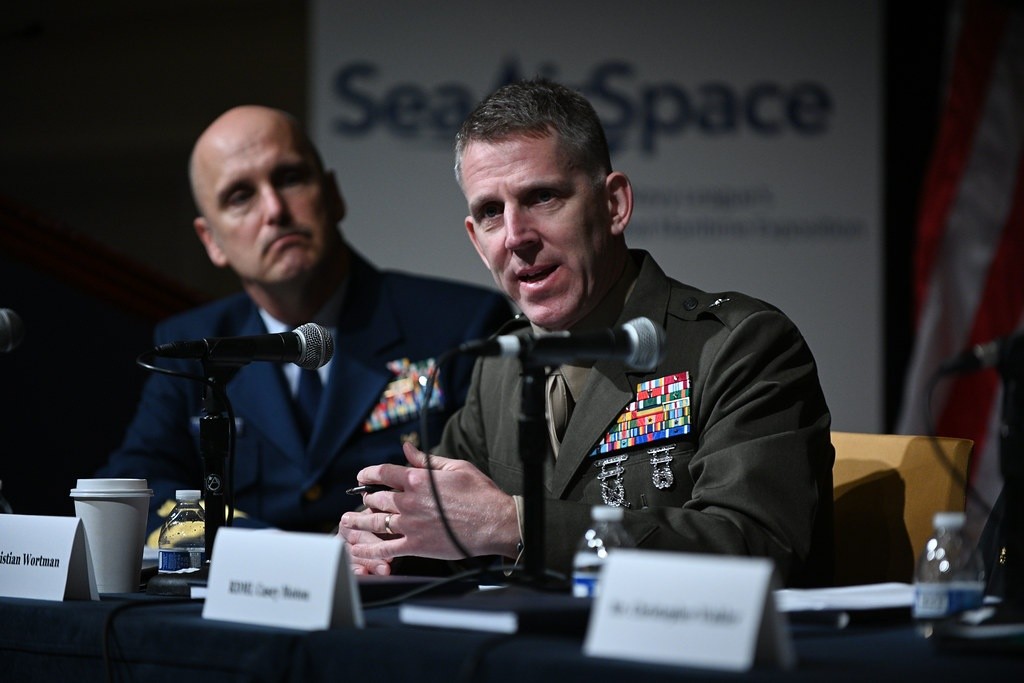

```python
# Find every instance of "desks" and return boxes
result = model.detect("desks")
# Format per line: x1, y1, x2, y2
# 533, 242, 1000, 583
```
0, 560, 1024, 683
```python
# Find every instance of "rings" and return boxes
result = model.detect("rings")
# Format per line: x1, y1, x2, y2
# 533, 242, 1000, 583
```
384, 514, 394, 535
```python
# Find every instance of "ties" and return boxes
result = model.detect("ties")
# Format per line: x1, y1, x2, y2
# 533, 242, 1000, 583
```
545, 368, 569, 461
295, 367, 324, 447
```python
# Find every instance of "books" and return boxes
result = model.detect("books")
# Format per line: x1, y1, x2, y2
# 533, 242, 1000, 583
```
397, 592, 594, 636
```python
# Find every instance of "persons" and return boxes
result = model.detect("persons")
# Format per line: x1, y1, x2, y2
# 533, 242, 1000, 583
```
93, 104, 515, 564
338, 77, 837, 608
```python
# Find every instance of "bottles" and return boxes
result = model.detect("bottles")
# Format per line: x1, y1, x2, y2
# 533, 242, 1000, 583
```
912, 511, 989, 643
571, 506, 634, 601
158, 490, 206, 575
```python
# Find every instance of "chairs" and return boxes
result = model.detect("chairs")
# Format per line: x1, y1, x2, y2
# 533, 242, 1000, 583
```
832, 430, 977, 586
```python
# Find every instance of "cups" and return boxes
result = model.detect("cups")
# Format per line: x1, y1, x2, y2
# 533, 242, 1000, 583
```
68, 478, 153, 601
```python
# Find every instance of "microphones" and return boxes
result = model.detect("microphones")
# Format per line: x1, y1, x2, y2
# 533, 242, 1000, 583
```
460, 316, 669, 373
0, 308, 21, 352
155, 322, 334, 372
932, 330, 1024, 379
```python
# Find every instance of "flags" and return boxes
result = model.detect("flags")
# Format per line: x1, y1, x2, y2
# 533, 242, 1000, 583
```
893, 0, 1024, 620
